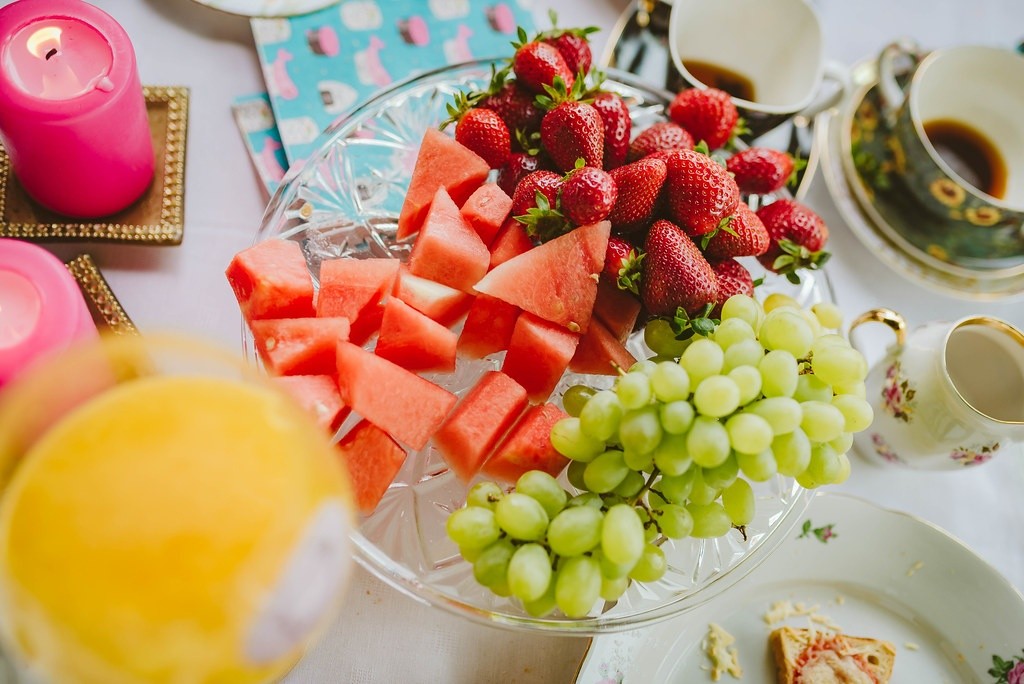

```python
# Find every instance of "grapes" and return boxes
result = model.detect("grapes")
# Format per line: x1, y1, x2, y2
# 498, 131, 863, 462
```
442, 292, 874, 618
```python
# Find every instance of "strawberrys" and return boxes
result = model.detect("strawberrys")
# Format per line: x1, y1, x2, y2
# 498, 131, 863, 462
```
434, 9, 833, 344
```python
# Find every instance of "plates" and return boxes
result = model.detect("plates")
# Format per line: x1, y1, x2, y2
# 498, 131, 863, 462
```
599, 0, 818, 206
572, 494, 1023, 684
843, 69, 1023, 279
243, 58, 843, 638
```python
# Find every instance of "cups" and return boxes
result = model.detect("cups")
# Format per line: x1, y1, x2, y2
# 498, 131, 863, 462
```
0, 334, 360, 684
876, 40, 1023, 228
669, 0, 847, 143
848, 307, 1024, 473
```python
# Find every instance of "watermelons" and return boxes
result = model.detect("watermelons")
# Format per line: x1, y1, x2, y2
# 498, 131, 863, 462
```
220, 125, 645, 518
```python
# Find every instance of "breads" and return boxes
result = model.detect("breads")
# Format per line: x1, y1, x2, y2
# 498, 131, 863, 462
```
771, 626, 896, 684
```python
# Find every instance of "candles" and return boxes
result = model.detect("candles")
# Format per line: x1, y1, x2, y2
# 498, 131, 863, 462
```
0, 0, 157, 219
0, 237, 100, 395
